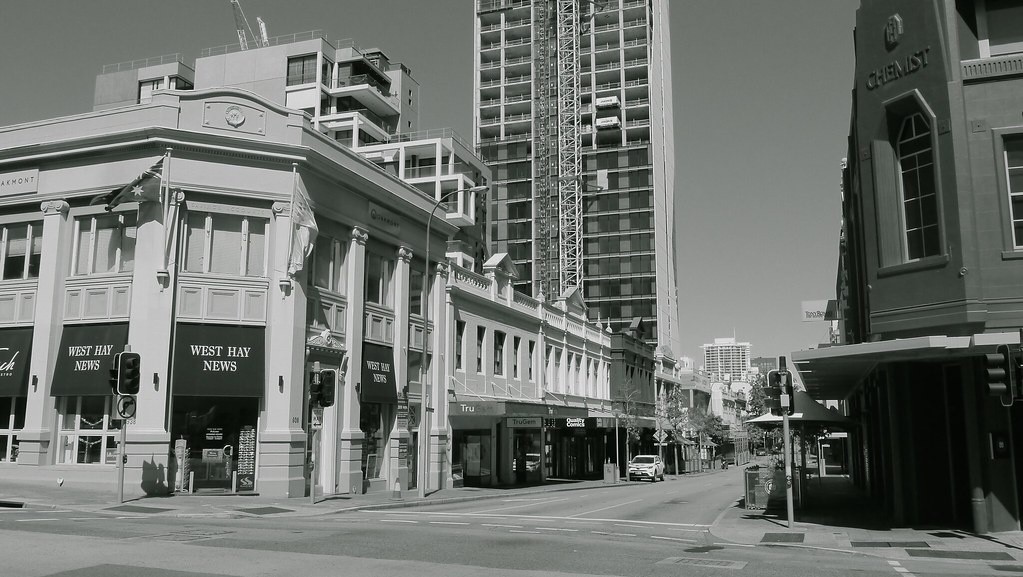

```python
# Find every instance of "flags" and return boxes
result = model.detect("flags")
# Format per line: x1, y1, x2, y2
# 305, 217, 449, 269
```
288, 186, 319, 273
103, 156, 165, 211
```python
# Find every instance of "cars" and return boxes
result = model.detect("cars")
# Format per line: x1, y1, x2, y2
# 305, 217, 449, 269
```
808, 456, 818, 463
709, 457, 728, 469
757, 448, 769, 456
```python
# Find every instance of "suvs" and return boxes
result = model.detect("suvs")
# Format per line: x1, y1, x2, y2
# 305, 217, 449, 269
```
628, 455, 666, 482
513, 454, 542, 471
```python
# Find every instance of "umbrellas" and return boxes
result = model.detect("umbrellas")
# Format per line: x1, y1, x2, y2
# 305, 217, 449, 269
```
746, 391, 850, 496
702, 439, 717, 446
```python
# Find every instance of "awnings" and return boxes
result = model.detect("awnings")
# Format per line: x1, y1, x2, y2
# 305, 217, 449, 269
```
0, 328, 33, 397
173, 323, 265, 397
671, 434, 693, 445
50, 323, 129, 396
361, 343, 397, 405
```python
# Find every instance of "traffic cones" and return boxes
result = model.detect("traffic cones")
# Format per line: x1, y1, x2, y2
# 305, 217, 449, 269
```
390, 479, 405, 502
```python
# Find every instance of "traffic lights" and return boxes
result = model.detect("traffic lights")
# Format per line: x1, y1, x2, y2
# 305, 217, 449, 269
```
764, 384, 781, 410
109, 353, 141, 395
311, 369, 335, 410
984, 343, 1014, 407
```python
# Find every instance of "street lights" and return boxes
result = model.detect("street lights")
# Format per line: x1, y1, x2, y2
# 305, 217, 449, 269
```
611, 409, 622, 484
418, 184, 491, 497
